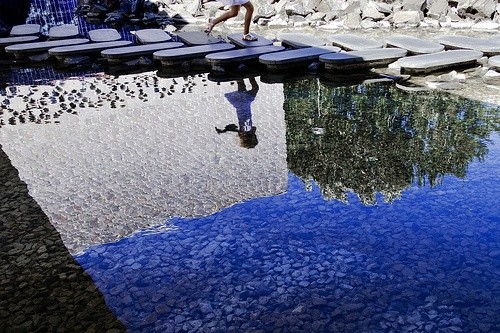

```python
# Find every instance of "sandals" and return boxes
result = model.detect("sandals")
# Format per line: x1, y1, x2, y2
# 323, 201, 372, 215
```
242, 33, 258, 41
205, 18, 214, 33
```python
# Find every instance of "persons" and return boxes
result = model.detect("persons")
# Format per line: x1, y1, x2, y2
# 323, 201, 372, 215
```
214, 78, 259, 149
204, 0, 258, 41
